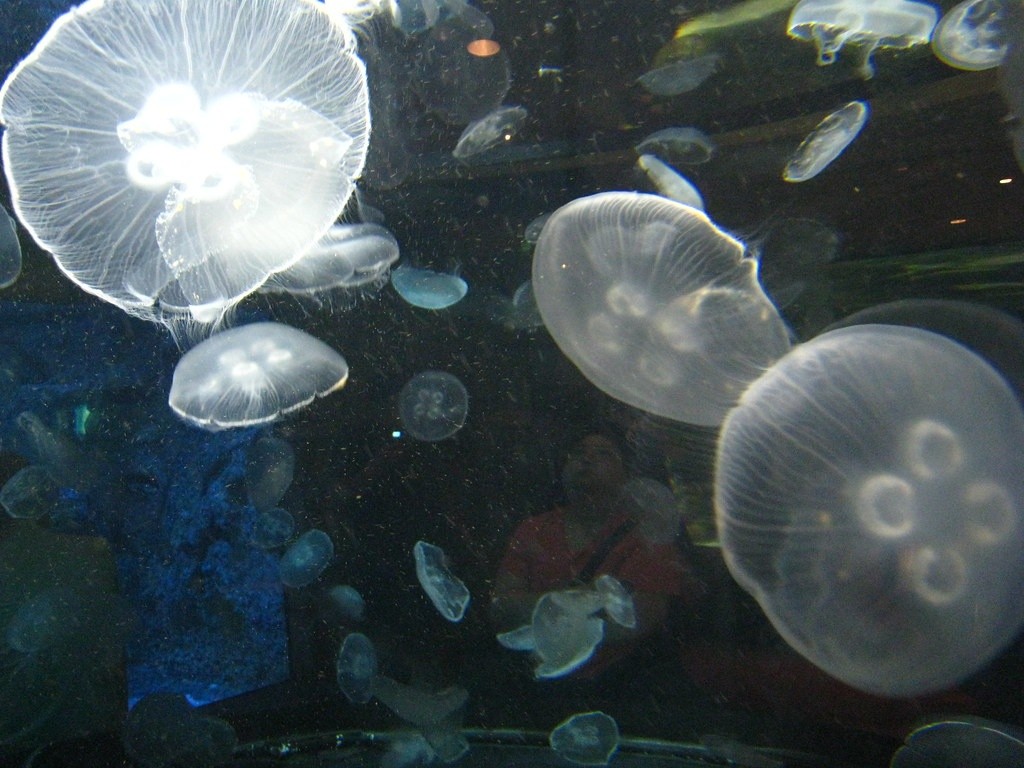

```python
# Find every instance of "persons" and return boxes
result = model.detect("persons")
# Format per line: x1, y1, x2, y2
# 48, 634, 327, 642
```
488, 432, 683, 768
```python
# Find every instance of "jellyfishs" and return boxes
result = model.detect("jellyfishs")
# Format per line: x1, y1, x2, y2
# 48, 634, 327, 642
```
0, 2, 1023, 767
